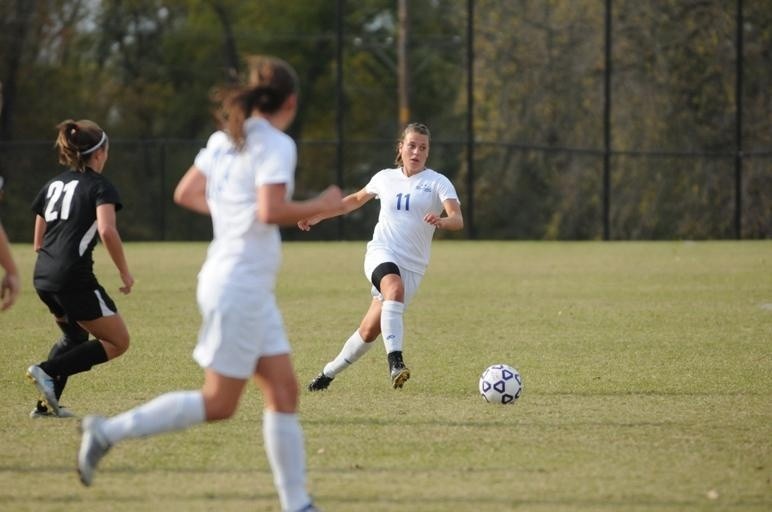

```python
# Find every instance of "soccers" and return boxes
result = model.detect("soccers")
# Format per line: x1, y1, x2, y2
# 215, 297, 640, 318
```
479, 365, 521, 403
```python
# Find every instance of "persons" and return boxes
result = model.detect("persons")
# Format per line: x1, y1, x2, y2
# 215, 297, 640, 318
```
28, 119, 134, 418
0, 224, 21, 310
297, 123, 465, 392
77, 55, 345, 510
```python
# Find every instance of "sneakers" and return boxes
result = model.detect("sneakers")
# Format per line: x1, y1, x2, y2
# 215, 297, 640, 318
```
27, 365, 60, 417
77, 413, 111, 487
29, 401, 73, 417
391, 362, 410, 388
308, 370, 335, 392
